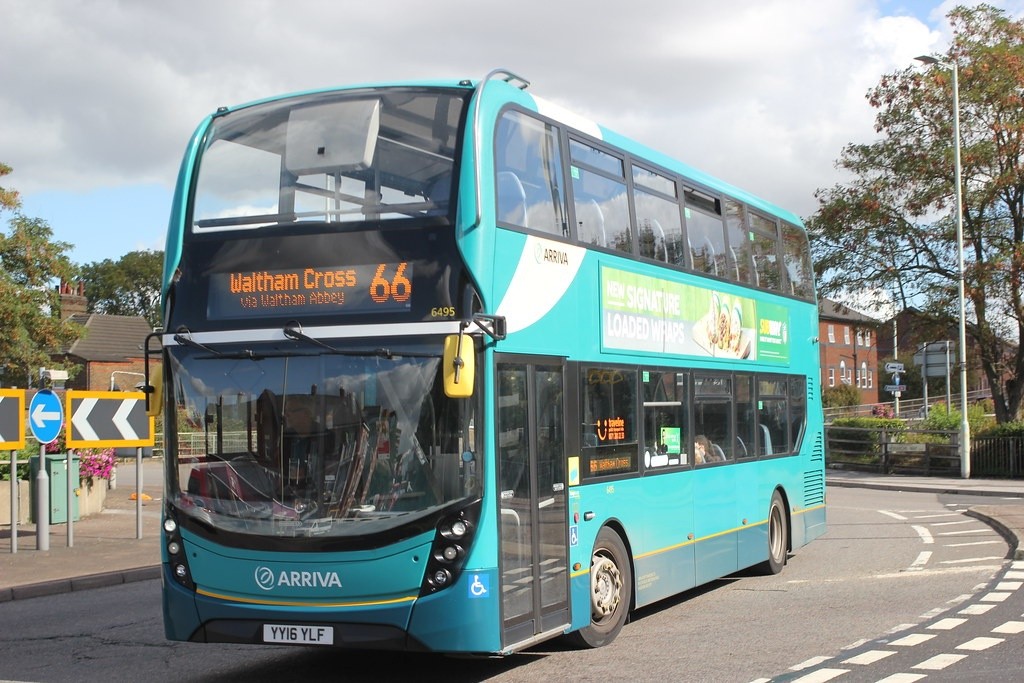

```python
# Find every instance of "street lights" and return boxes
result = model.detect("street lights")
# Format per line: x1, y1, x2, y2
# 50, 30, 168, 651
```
914, 55, 970, 477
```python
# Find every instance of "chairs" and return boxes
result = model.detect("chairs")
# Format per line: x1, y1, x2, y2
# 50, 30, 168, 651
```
707, 414, 793, 460
428, 170, 794, 297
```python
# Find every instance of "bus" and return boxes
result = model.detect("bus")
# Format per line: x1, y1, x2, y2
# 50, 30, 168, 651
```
133, 67, 828, 660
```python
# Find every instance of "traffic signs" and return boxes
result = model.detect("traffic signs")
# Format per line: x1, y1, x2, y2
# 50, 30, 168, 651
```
884, 361, 908, 374
884, 385, 907, 393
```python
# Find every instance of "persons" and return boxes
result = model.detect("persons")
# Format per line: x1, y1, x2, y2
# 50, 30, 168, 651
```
694, 433, 721, 465
266, 395, 335, 504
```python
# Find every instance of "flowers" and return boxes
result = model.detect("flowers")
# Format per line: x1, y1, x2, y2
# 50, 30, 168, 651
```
44, 422, 116, 485
